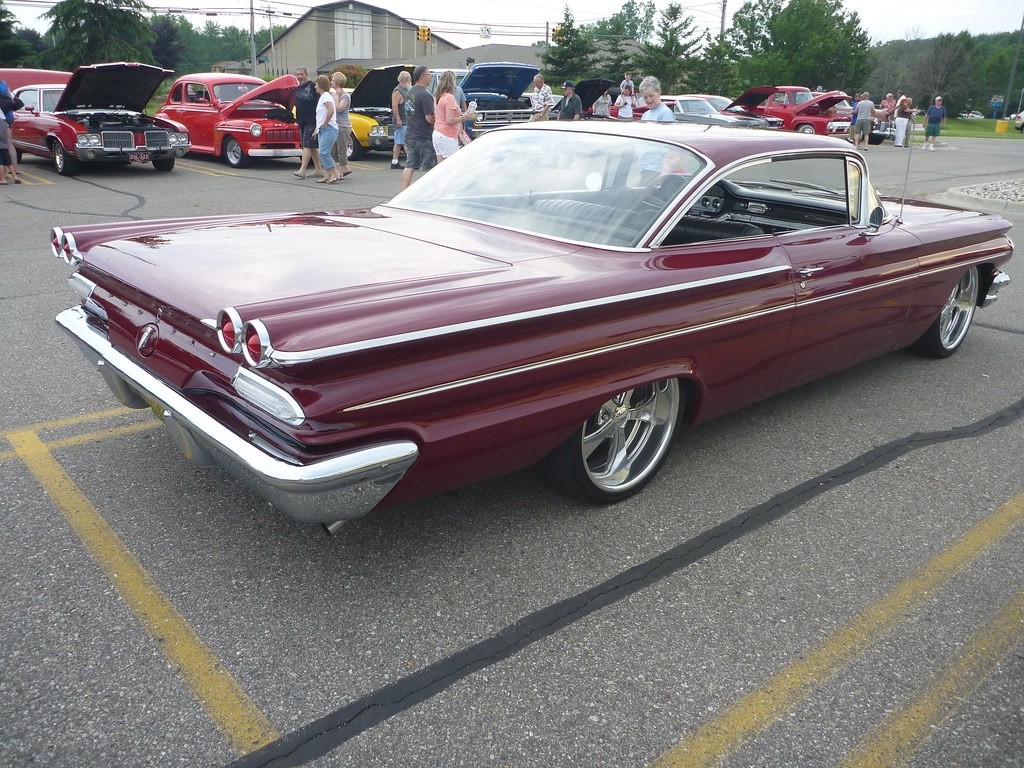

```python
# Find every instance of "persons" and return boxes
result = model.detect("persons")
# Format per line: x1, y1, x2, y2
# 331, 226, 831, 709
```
391, 71, 412, 169
557, 81, 582, 120
432, 71, 477, 165
0, 80, 24, 185
311, 75, 342, 184
922, 96, 946, 151
530, 74, 554, 122
403, 66, 436, 189
638, 76, 676, 188
614, 72, 637, 120
293, 66, 324, 178
330, 72, 353, 180
846, 90, 917, 150
592, 89, 612, 118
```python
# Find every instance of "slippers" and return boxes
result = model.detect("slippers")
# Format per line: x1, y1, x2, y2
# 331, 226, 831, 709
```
337, 165, 352, 176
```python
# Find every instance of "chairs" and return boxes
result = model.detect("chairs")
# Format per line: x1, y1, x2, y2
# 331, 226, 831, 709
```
195, 91, 210, 103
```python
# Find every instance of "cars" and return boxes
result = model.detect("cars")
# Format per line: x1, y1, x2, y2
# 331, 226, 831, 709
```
966, 111, 984, 120
500, 78, 616, 120
279, 64, 418, 161
49, 120, 1013, 537
796, 92, 896, 145
425, 61, 540, 137
1015, 111, 1024, 134
659, 95, 769, 130
586, 101, 675, 121
0, 62, 193, 176
153, 72, 314, 168
677, 85, 785, 131
958, 110, 966, 119
741, 86, 854, 143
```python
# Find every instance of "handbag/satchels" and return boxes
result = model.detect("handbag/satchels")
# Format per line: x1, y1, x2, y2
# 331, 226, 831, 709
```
0, 92, 24, 115
458, 122, 472, 146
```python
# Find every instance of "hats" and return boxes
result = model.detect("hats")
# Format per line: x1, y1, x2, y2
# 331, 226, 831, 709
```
935, 96, 943, 101
560, 80, 573, 88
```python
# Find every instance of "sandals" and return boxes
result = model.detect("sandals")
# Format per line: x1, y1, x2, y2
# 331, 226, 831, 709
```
317, 176, 331, 183
326, 172, 342, 183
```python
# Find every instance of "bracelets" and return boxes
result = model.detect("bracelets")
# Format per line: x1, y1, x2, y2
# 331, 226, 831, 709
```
540, 113, 543, 116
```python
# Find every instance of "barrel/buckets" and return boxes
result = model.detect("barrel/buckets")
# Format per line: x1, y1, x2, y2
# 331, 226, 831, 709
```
995, 120, 1008, 134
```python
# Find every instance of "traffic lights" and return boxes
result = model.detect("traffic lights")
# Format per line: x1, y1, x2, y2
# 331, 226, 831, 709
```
552, 29, 557, 41
559, 34, 563, 42
417, 27, 423, 41
425, 28, 431, 42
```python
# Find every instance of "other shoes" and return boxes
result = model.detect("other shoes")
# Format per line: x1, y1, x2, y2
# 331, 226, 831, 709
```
336, 173, 344, 180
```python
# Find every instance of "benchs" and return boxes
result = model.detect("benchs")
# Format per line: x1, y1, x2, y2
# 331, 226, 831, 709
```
641, 210, 764, 248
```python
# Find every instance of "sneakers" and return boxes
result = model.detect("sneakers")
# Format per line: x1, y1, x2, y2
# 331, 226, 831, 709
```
294, 170, 306, 178
922, 144, 926, 149
391, 162, 405, 169
929, 146, 935, 152
306, 170, 324, 178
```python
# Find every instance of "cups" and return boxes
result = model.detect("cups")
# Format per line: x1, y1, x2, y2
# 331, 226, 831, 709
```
466, 100, 478, 112
